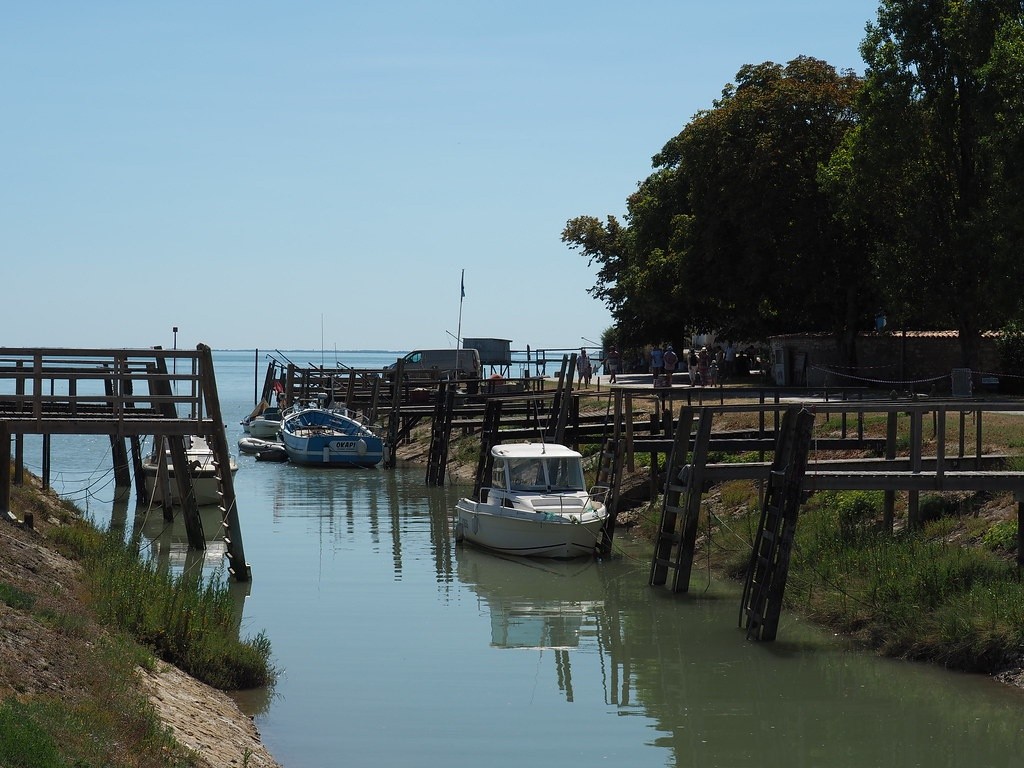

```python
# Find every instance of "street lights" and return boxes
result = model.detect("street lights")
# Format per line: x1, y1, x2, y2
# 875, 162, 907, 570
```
172, 326, 178, 385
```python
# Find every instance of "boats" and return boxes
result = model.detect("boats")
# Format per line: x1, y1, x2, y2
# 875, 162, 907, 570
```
141, 434, 238, 504
454, 392, 611, 560
249, 416, 281, 438
264, 407, 282, 422
239, 415, 265, 433
238, 437, 287, 454
278, 393, 384, 468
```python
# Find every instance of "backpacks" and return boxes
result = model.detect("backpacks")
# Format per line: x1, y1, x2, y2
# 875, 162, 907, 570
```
691, 353, 697, 366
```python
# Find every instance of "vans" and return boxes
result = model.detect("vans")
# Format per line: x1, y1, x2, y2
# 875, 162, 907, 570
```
386, 349, 481, 387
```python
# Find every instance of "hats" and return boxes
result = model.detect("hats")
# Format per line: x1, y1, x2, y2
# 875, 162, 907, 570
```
701, 347, 707, 352
667, 346, 673, 351
711, 360, 717, 364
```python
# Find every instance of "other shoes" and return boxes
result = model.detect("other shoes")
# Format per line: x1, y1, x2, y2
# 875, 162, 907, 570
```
692, 380, 695, 387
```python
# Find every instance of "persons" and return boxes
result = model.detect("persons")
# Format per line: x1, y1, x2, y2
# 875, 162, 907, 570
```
687, 339, 737, 387
601, 345, 621, 384
577, 350, 591, 390
650, 346, 678, 387
